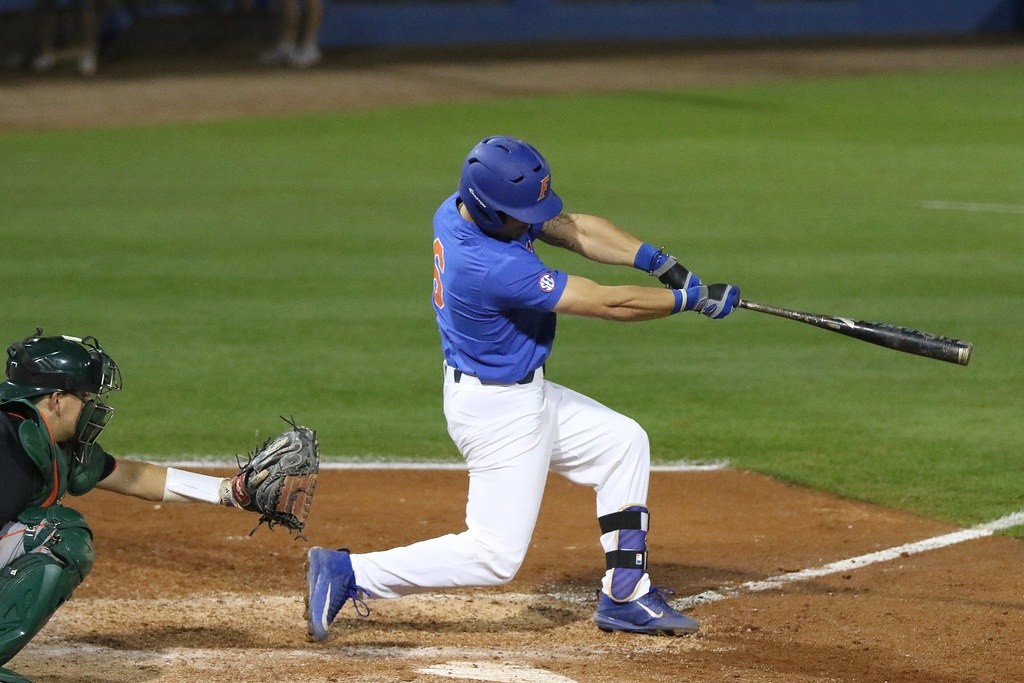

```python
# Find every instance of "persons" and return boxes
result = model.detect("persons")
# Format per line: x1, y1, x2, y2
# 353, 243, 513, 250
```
304, 136, 741, 643
269, 0, 323, 68
0, 328, 320, 683
29, 0, 102, 77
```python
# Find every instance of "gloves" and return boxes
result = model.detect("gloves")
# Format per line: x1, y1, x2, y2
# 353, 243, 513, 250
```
670, 283, 742, 321
634, 241, 702, 289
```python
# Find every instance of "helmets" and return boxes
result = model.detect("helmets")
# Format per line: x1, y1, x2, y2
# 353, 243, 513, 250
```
6, 335, 112, 393
457, 135, 564, 233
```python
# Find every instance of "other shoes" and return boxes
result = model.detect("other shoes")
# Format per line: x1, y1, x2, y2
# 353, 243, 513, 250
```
30, 50, 56, 77
70, 47, 99, 77
275, 40, 322, 69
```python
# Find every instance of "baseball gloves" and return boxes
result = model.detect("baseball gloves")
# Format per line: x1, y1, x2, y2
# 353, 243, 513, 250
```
216, 422, 319, 531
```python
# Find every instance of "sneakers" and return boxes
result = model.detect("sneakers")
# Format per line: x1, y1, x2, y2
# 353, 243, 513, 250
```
307, 545, 373, 640
595, 585, 699, 636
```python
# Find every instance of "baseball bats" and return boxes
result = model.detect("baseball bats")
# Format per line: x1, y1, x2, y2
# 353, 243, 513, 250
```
740, 299, 972, 367
